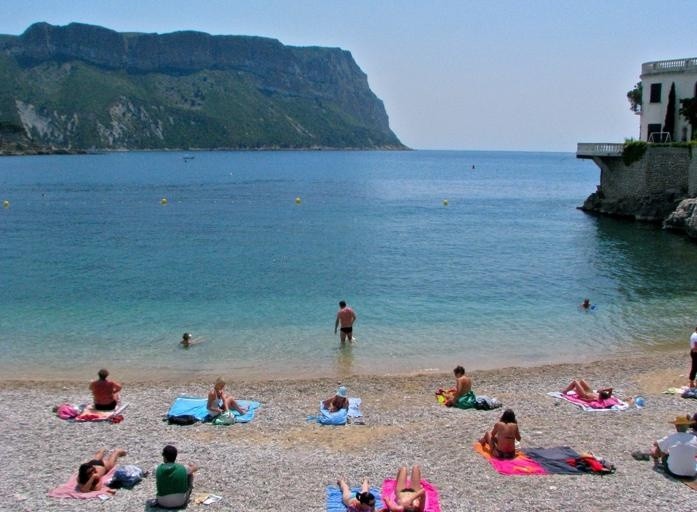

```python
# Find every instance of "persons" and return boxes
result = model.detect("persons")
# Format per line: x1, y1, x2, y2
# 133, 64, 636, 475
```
437, 365, 472, 404
321, 385, 349, 412
382, 464, 426, 511
556, 379, 613, 403
154, 444, 200, 510
88, 369, 122, 411
76, 446, 128, 492
648, 413, 695, 482
579, 296, 593, 309
336, 476, 377, 511
686, 326, 696, 387
333, 300, 357, 343
205, 376, 250, 417
477, 407, 521, 459
179, 330, 209, 349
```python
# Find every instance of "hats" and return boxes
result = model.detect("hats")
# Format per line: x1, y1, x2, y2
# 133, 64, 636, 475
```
336, 387, 349, 399
667, 415, 697, 426
